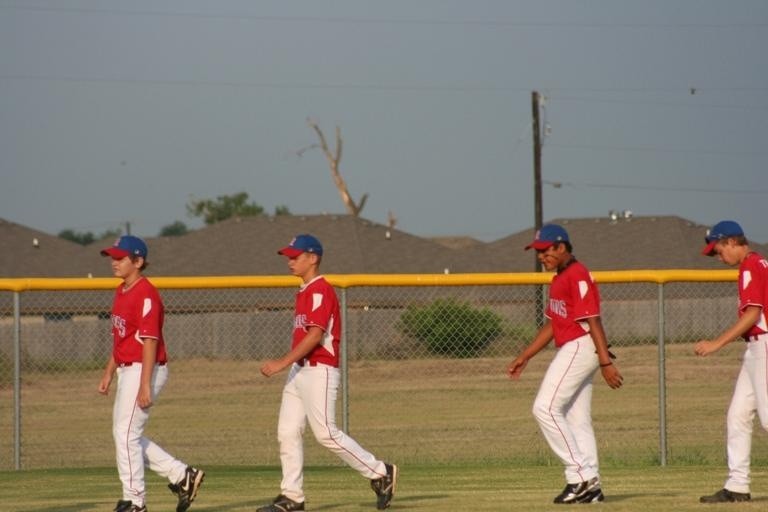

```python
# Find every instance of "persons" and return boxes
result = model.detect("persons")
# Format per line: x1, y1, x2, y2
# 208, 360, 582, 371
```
506, 224, 623, 504
98, 235, 204, 512
257, 234, 398, 512
691, 221, 767, 503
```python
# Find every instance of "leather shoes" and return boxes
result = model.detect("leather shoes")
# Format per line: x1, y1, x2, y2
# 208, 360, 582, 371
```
699, 488, 752, 504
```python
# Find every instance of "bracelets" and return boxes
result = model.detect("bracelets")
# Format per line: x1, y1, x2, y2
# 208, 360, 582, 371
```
598, 362, 613, 367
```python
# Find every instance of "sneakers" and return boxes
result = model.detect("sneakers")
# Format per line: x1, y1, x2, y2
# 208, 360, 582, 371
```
112, 498, 148, 512
569, 485, 605, 505
256, 493, 305, 512
554, 478, 599, 504
372, 462, 397, 509
167, 464, 205, 512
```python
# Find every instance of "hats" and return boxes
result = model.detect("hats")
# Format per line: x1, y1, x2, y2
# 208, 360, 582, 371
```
100, 235, 148, 263
277, 234, 324, 259
701, 219, 743, 257
525, 224, 570, 251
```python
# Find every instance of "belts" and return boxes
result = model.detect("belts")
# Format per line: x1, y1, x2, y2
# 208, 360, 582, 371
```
742, 333, 760, 343
298, 358, 318, 368
115, 361, 168, 368
